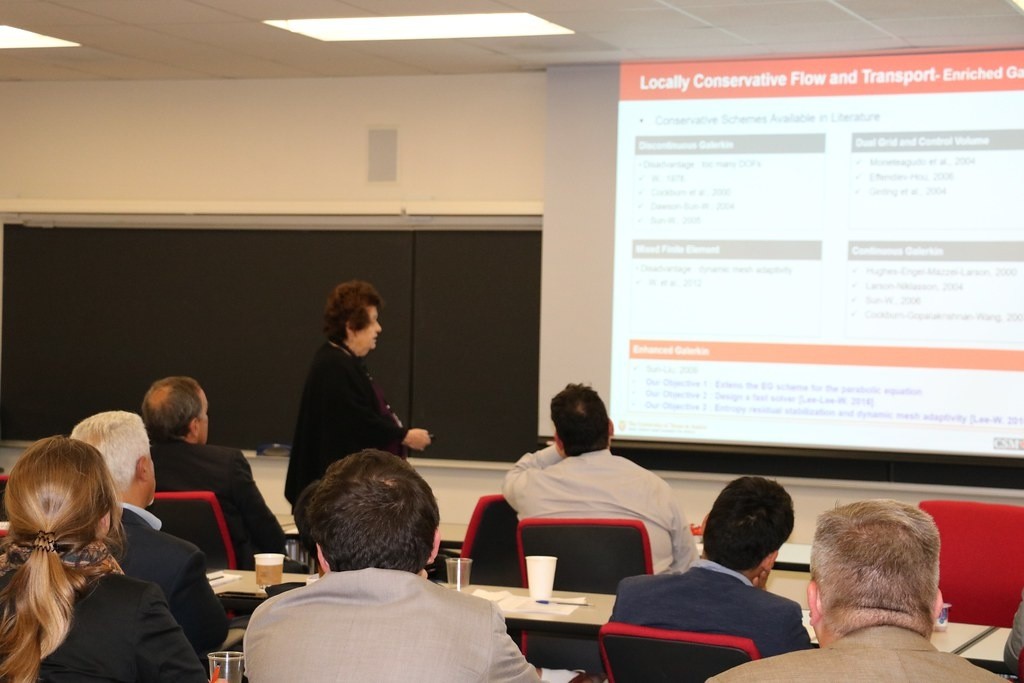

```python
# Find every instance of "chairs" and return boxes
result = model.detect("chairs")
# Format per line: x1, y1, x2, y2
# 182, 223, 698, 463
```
143, 492, 1024, 683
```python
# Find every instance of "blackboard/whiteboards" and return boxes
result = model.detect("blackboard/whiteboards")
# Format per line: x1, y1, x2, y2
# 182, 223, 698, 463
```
1, 212, 1024, 501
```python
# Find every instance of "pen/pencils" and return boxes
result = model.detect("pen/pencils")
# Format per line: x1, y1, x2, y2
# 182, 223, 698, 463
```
209, 575, 224, 582
536, 600, 590, 606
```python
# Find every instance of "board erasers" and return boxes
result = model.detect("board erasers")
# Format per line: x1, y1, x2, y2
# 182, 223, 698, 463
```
255, 443, 293, 457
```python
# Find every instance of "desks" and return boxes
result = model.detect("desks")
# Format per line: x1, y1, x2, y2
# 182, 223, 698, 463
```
955, 627, 1012, 668
690, 538, 810, 572
203, 565, 461, 611
267, 513, 470, 570
440, 584, 995, 659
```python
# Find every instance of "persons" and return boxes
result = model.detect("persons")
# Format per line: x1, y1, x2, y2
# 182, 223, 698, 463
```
0, 436, 211, 682
1004, 588, 1024, 683
501, 383, 699, 575
285, 282, 431, 515
242, 448, 607, 683
142, 377, 309, 574
706, 498, 1013, 683
70, 409, 229, 657
608, 478, 813, 658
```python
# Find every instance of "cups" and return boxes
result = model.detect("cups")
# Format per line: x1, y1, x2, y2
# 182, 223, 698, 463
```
525, 555, 558, 602
254, 553, 286, 594
446, 558, 473, 594
207, 651, 245, 683
934, 604, 953, 631
